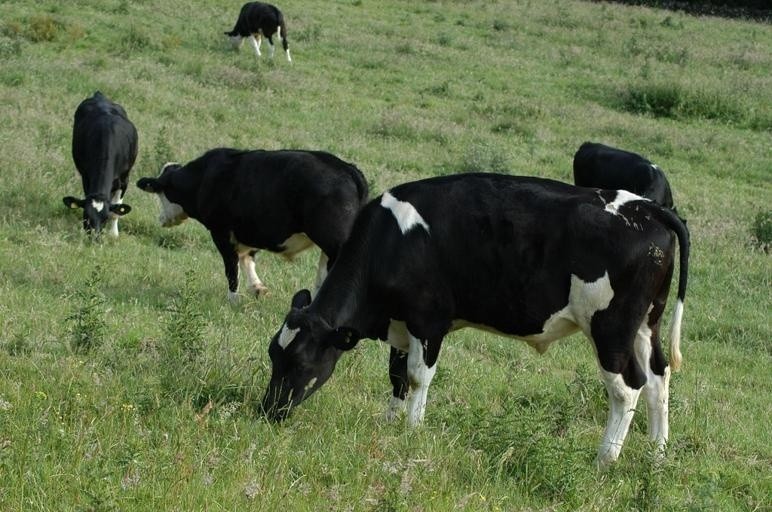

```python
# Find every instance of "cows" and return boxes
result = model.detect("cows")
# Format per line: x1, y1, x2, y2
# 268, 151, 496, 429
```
573, 141, 689, 226
258, 172, 690, 469
223, 1, 293, 67
63, 91, 138, 252
135, 148, 370, 308
